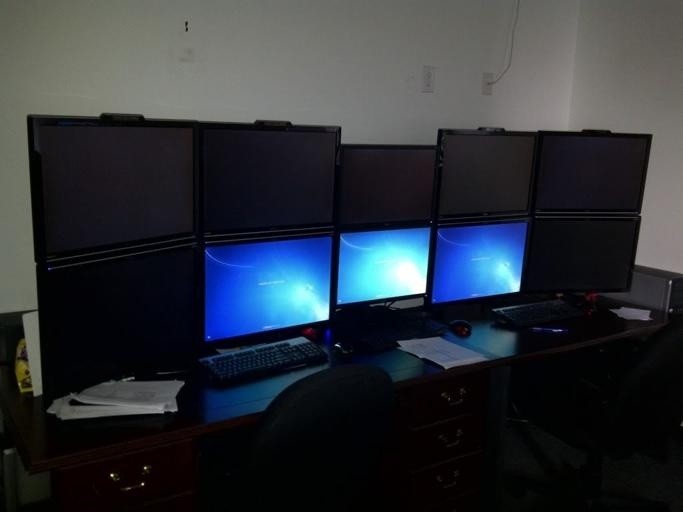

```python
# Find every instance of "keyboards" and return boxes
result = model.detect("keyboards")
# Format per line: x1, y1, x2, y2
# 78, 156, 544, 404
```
198, 335, 328, 388
490, 298, 579, 331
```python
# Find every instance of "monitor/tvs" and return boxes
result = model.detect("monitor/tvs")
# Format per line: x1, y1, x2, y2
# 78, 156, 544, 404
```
197, 230, 335, 336
436, 126, 537, 214
337, 225, 435, 320
339, 142, 438, 224
534, 130, 654, 215
198, 123, 341, 242
431, 215, 532, 308
35, 237, 202, 411
526, 211, 640, 295
27, 113, 199, 263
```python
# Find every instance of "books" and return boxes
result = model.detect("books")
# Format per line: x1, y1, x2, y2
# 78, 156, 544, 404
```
397, 335, 490, 370
47, 378, 188, 422
610, 300, 654, 324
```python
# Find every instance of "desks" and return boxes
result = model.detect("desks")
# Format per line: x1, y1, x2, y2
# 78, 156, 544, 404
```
0, 295, 668, 511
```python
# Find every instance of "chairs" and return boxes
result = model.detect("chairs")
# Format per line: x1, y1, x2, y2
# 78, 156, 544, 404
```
206, 363, 397, 510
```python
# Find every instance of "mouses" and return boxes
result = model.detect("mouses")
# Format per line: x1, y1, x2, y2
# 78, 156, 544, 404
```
450, 315, 473, 339
333, 334, 351, 355
